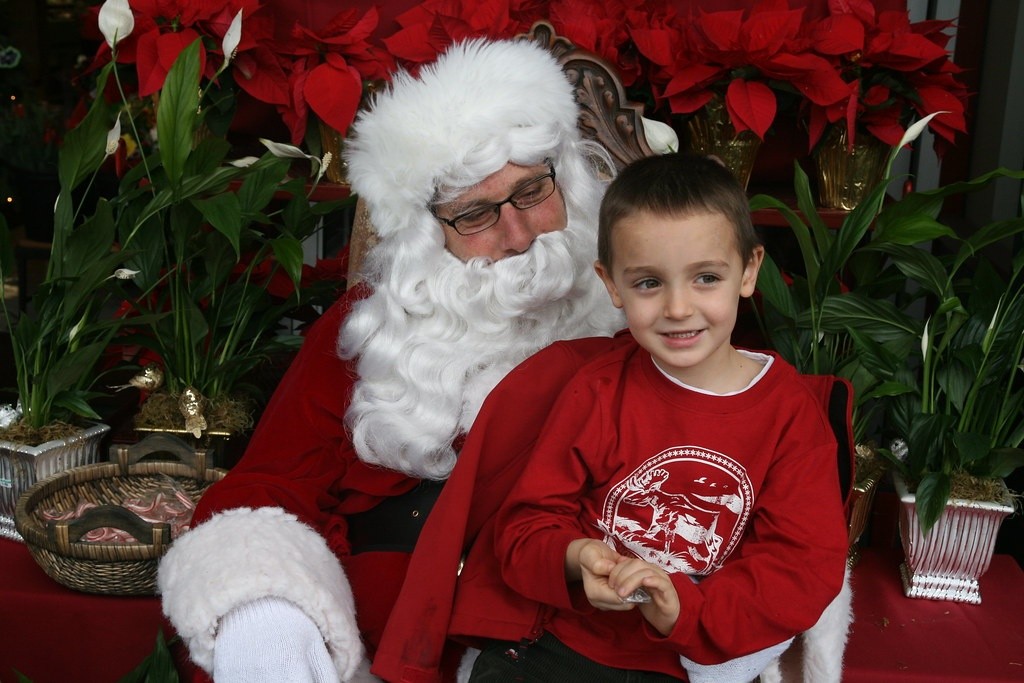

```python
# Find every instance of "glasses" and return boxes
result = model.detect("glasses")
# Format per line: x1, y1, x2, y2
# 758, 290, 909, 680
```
432, 159, 556, 236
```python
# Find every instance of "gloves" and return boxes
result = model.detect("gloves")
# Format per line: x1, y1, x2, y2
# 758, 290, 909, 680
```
212, 595, 339, 683
679, 635, 796, 683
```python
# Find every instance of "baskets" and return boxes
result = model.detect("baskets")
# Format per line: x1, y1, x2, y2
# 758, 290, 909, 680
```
13, 446, 230, 595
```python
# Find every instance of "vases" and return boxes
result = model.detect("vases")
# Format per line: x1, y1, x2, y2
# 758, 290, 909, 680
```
134, 426, 231, 470
0, 420, 111, 542
847, 471, 877, 570
890, 469, 1013, 605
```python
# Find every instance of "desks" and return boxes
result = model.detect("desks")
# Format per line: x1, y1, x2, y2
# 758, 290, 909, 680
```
0, 545, 1024, 683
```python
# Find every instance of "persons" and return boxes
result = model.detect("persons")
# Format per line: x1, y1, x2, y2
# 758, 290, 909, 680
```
470, 152, 848, 683
156, 35, 854, 683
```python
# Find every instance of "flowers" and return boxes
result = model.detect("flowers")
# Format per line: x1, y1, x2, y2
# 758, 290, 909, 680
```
0, 0, 1024, 545
